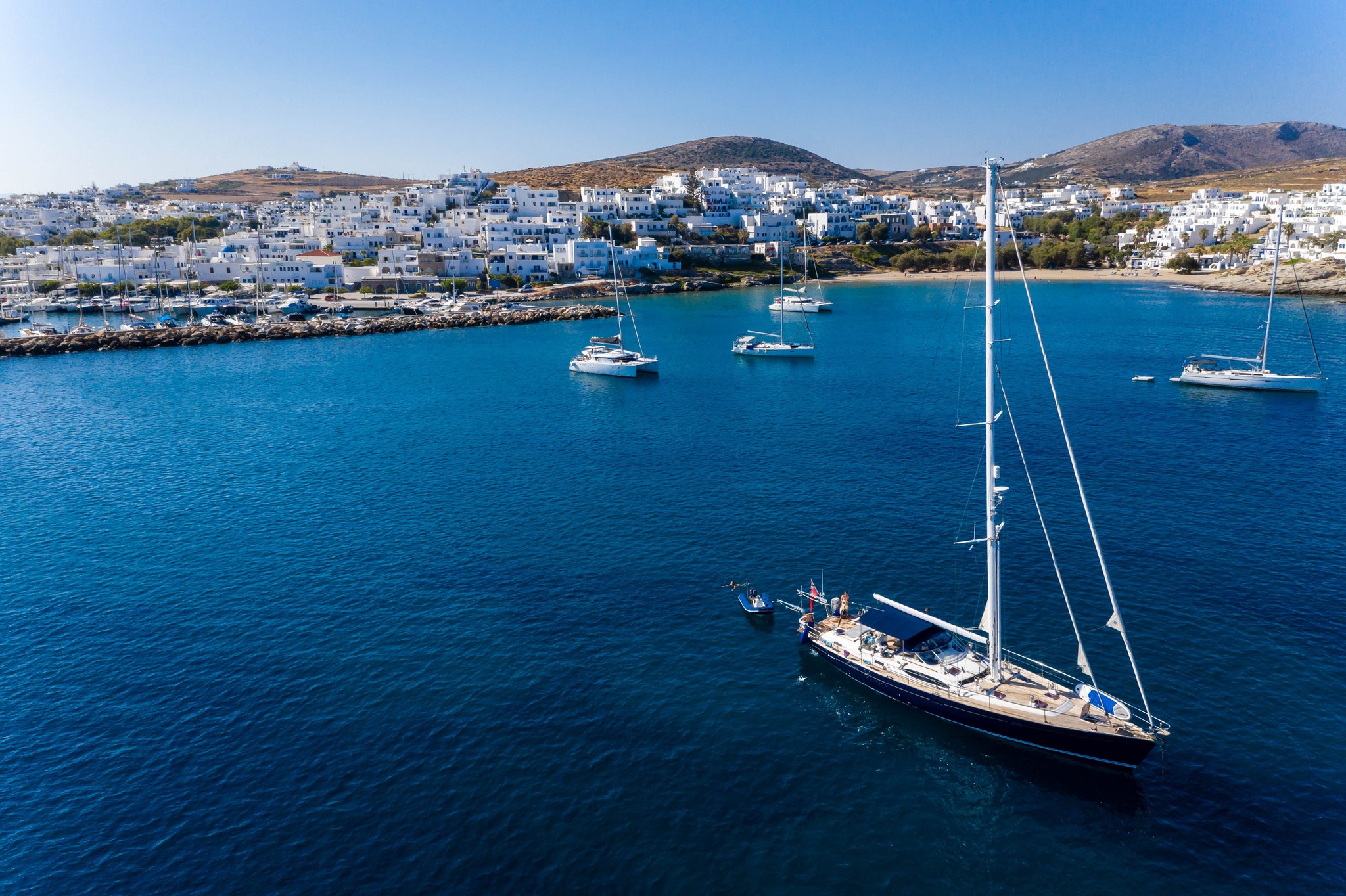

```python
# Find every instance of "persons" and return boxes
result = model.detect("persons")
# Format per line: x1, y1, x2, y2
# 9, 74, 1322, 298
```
840, 591, 849, 619
731, 581, 735, 587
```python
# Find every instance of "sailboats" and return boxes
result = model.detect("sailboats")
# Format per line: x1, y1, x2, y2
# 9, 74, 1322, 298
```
0, 226, 488, 336
568, 223, 660, 378
766, 207, 835, 313
1178, 204, 1324, 394
732, 226, 819, 359
797, 157, 1172, 769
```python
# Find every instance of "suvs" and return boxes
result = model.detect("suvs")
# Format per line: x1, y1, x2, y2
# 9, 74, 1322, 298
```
518, 284, 533, 293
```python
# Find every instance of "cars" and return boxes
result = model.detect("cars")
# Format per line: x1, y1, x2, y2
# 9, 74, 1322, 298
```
807, 241, 819, 247
903, 239, 908, 243
916, 239, 921, 242
935, 237, 942, 242
819, 242, 826, 247
837, 241, 846, 245
926, 238, 933, 242
1040, 233, 1045, 237
876, 240, 885, 244
826, 241, 834, 246
796, 242, 803, 246
791, 244, 795, 248
866, 240, 875, 245
886, 239, 895, 244
847, 241, 856, 245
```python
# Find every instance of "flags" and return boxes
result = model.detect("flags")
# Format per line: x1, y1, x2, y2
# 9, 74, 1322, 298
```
810, 580, 818, 613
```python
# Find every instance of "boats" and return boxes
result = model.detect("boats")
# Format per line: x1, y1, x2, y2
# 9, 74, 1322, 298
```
738, 592, 775, 618
1130, 375, 1156, 381
